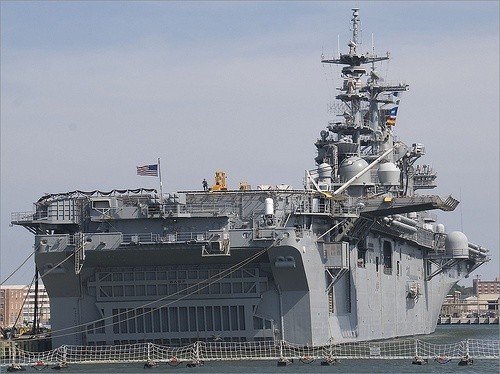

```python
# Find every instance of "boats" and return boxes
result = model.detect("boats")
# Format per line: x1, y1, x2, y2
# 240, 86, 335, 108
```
12, 259, 53, 357
440, 294, 500, 325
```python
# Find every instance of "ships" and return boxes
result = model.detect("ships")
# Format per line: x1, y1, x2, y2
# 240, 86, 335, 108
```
9, 6, 490, 353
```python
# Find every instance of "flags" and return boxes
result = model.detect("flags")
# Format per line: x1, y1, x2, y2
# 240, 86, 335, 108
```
388, 107, 397, 116
386, 117, 396, 126
136, 164, 158, 176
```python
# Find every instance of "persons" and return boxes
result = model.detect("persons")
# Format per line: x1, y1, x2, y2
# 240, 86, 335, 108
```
202, 179, 208, 191
239, 183, 244, 190
